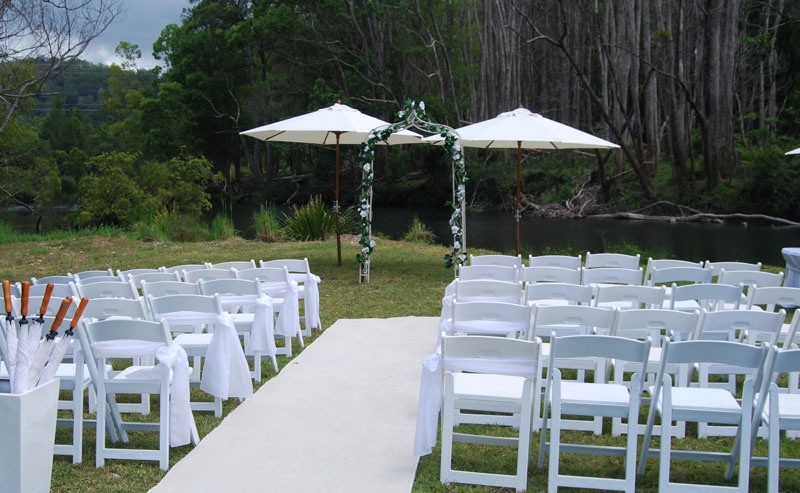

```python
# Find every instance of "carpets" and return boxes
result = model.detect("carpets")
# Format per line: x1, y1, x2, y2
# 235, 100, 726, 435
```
146, 316, 445, 493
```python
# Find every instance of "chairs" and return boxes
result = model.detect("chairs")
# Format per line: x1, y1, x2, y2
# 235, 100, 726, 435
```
1, 257, 322, 470
441, 252, 800, 493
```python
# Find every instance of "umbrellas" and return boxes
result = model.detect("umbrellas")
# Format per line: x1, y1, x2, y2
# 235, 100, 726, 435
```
422, 103, 620, 257
238, 100, 425, 267
0, 280, 89, 395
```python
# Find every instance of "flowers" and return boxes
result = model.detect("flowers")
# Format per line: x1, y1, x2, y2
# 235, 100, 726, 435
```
356, 99, 466, 270
